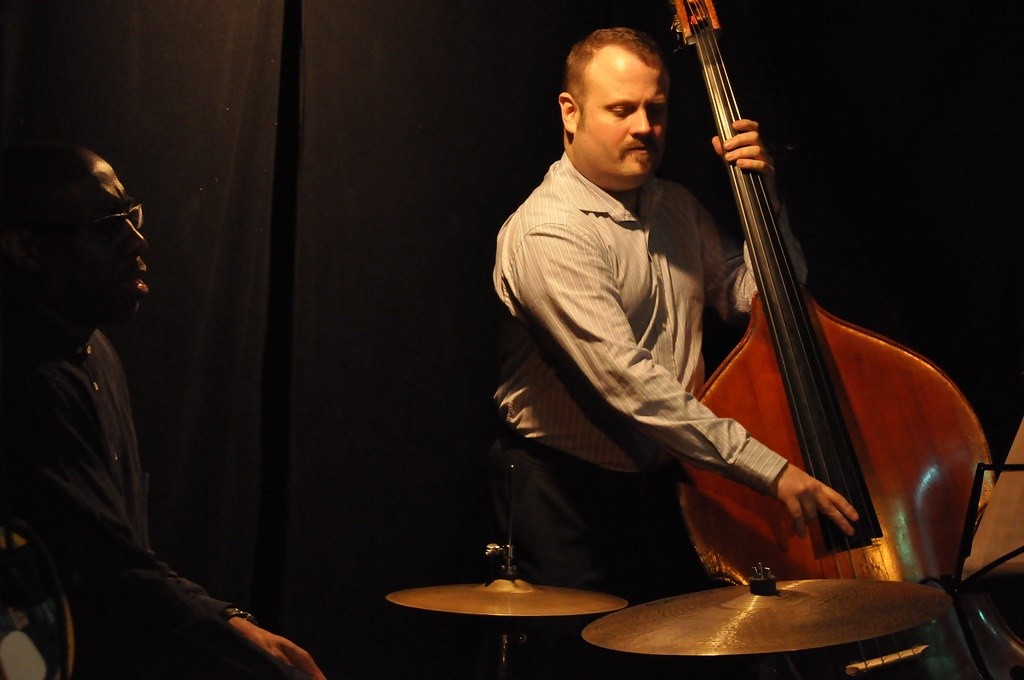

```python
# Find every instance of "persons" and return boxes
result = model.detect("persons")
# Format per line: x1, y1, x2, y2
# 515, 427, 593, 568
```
491, 24, 860, 584
0, 139, 326, 680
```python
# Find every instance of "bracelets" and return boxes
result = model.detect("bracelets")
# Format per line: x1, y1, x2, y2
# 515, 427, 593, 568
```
222, 607, 260, 625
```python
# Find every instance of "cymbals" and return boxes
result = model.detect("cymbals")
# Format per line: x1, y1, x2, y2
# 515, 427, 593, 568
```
384, 577, 628, 619
580, 578, 954, 658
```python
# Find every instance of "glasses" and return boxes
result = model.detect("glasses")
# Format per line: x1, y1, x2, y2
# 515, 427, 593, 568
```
7, 203, 143, 243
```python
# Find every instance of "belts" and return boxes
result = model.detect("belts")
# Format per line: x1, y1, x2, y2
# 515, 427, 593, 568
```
497, 418, 628, 494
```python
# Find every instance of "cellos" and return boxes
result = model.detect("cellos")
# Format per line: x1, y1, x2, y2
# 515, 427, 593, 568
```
671, 2, 1024, 680
